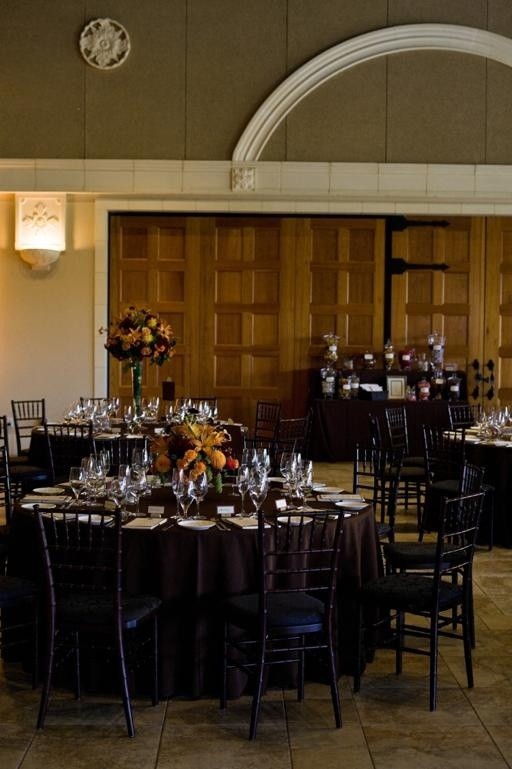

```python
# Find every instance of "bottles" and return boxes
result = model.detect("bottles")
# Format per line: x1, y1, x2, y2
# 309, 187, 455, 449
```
446, 373, 461, 403
316, 365, 361, 401
416, 353, 430, 370
383, 342, 395, 369
397, 344, 416, 370
426, 331, 447, 370
322, 331, 341, 367
418, 376, 431, 401
432, 371, 448, 400
361, 349, 377, 370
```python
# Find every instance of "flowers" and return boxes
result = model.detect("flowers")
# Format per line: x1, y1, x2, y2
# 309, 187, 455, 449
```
96, 304, 180, 369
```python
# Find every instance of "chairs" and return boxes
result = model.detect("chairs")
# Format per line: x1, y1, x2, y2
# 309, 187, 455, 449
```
31, 505, 166, 739
365, 490, 488, 713
0, 393, 312, 508
207, 504, 351, 742
351, 404, 494, 548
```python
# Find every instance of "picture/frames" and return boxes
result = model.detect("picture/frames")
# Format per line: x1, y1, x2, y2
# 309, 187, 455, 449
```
386, 375, 407, 401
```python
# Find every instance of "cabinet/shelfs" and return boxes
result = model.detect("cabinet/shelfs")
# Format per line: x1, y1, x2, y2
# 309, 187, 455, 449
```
307, 363, 477, 464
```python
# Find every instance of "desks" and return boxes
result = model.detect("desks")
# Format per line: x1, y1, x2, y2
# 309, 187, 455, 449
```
443, 424, 510, 548
30, 418, 243, 487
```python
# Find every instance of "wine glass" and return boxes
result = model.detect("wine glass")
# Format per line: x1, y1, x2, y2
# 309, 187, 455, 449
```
472, 402, 512, 442
64, 445, 316, 516
64, 395, 212, 435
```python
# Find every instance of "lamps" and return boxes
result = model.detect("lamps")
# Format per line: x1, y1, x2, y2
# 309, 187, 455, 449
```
19, 244, 62, 272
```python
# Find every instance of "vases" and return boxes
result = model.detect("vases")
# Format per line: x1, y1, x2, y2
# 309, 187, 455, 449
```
128, 360, 147, 418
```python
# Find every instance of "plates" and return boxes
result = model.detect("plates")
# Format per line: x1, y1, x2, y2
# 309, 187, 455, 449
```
336, 500, 368, 511
181, 520, 218, 532
275, 515, 315, 525
33, 486, 62, 496
314, 485, 345, 495
20, 502, 57, 511
